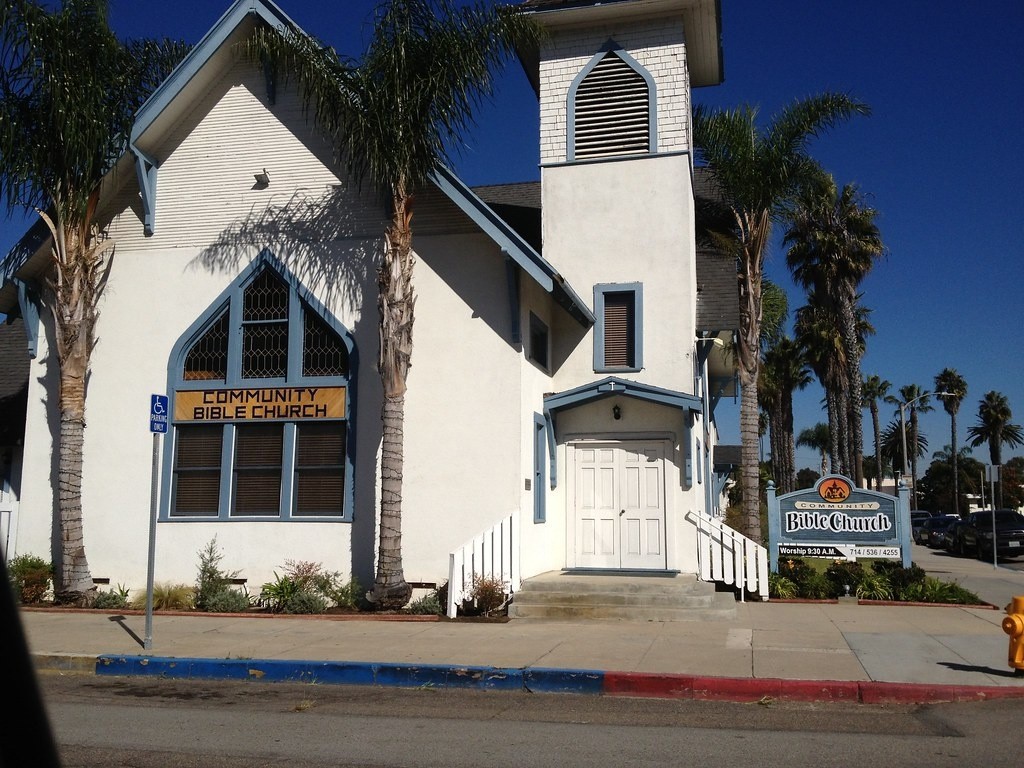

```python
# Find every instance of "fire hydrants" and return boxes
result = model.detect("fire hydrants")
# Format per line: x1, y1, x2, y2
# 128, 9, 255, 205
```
1001, 594, 1024, 678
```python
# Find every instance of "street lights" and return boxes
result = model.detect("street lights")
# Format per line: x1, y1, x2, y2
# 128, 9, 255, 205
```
900, 391, 948, 474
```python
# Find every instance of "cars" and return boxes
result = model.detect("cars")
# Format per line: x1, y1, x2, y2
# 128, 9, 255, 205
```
944, 509, 1024, 562
916, 516, 961, 550
910, 509, 933, 543
